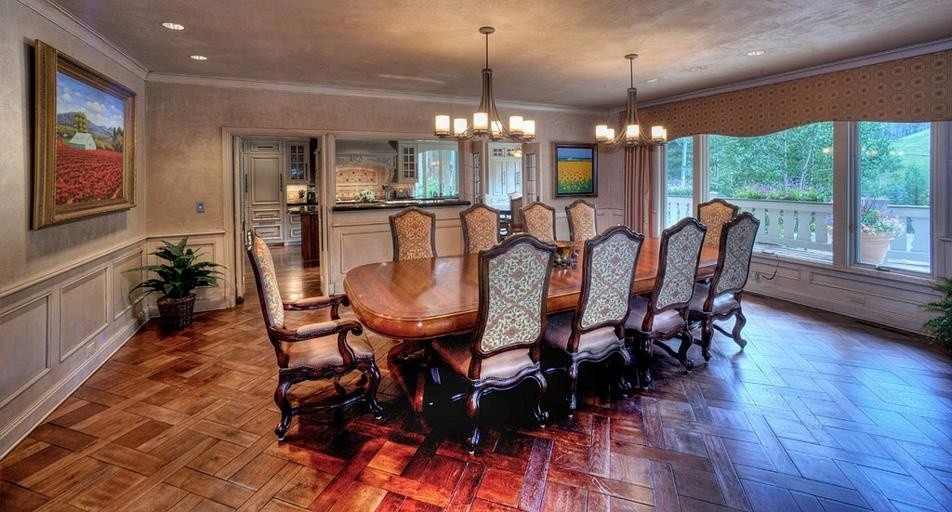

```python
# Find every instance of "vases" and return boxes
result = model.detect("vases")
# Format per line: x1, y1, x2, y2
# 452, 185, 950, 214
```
855, 228, 891, 266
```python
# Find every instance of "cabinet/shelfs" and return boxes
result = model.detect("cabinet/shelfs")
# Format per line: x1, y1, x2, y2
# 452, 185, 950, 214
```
390, 140, 420, 185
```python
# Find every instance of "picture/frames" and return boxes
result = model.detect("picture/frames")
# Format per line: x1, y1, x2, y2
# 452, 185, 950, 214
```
32, 39, 139, 228
548, 142, 602, 199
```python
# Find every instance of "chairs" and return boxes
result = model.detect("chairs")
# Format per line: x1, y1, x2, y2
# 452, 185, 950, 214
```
387, 205, 436, 258
518, 201, 559, 242
693, 209, 761, 362
424, 235, 552, 451
461, 205, 500, 252
632, 216, 706, 380
530, 226, 645, 421
247, 232, 396, 456
565, 200, 601, 241
696, 197, 742, 248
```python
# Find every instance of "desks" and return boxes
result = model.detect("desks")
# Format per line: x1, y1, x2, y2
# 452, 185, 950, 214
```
345, 235, 720, 423
285, 206, 321, 266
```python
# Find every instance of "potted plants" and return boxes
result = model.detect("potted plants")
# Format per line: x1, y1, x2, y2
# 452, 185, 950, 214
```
126, 235, 228, 332
920, 272, 952, 357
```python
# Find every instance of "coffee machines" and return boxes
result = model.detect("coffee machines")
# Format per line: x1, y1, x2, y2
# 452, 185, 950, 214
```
306, 184, 316, 203
302, 205, 318, 213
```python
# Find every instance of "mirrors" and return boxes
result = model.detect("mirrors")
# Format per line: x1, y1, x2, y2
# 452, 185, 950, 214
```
332, 138, 465, 202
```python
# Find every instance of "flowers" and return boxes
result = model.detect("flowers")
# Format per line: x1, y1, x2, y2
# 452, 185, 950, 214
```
731, 178, 829, 202
667, 175, 694, 196
856, 203, 904, 237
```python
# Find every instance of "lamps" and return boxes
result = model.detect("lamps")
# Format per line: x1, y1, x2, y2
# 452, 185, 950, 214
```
595, 54, 669, 148
431, 26, 535, 142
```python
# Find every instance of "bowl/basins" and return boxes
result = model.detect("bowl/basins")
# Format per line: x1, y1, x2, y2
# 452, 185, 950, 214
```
548, 242, 579, 267
